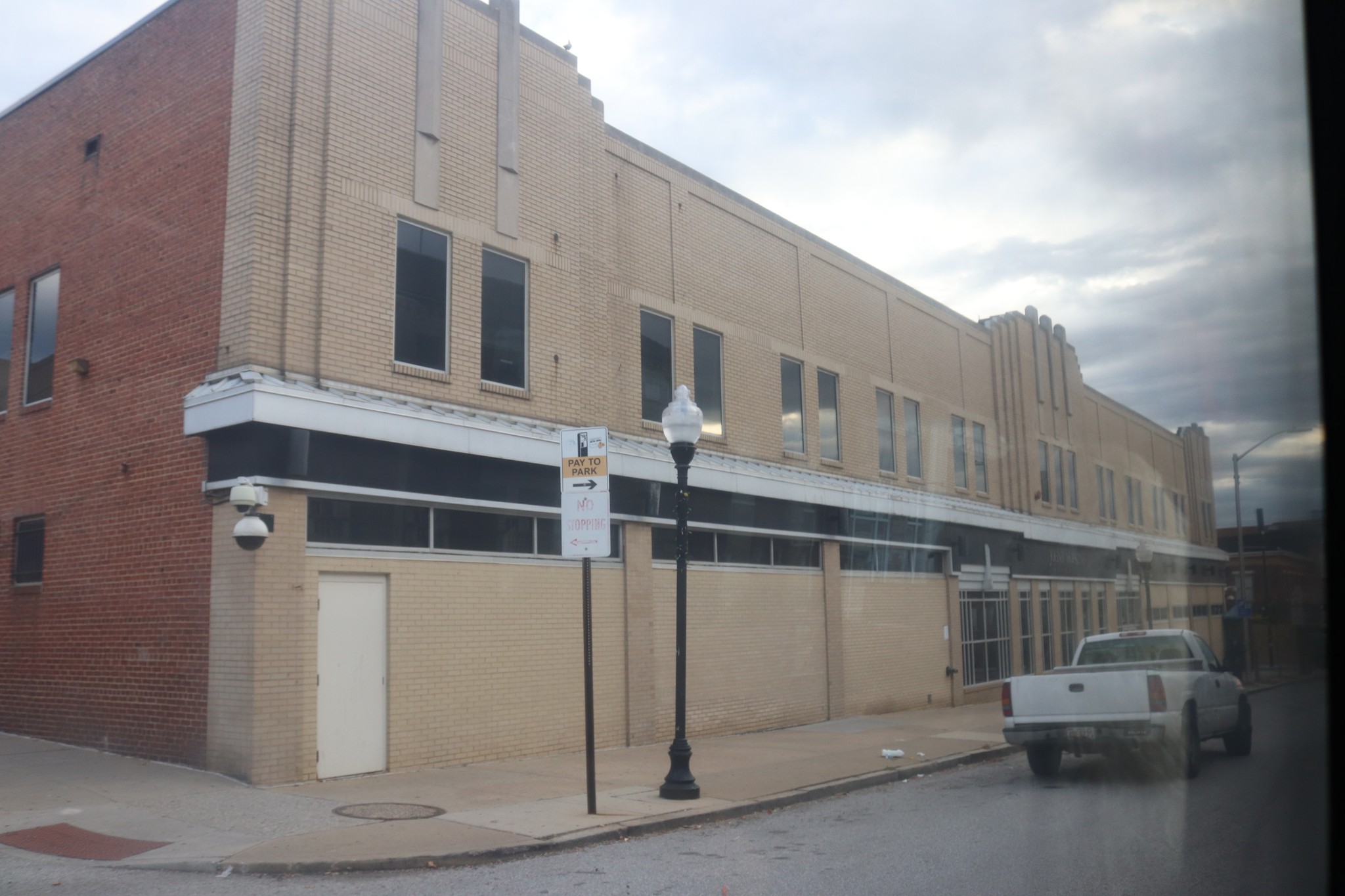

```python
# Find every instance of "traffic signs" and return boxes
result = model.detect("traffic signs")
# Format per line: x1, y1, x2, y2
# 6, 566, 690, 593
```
562, 456, 612, 559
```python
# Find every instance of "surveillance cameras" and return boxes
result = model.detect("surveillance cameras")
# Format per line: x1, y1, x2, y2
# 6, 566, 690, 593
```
232, 513, 268, 552
229, 485, 256, 513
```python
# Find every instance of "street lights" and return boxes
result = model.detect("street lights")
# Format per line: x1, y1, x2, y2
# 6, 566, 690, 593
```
660, 384, 705, 800
1231, 428, 1314, 680
1135, 540, 1153, 629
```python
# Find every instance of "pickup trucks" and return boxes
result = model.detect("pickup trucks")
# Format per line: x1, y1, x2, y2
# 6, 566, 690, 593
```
999, 629, 1255, 779
